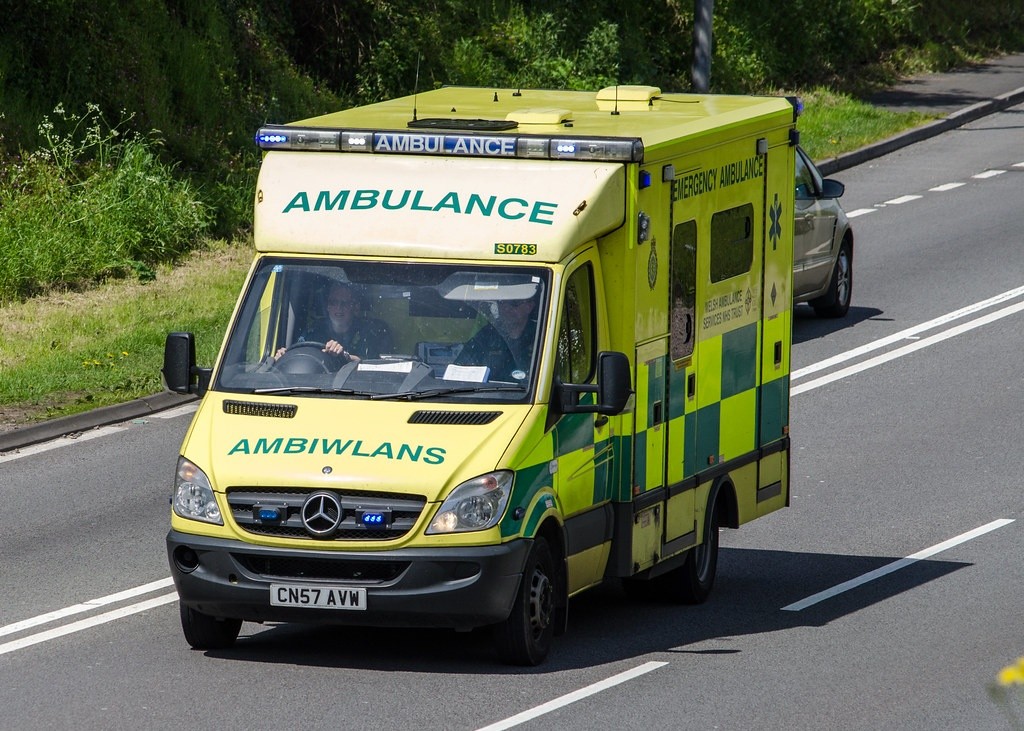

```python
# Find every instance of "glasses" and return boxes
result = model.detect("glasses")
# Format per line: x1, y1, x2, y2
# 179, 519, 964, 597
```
327, 298, 356, 306
499, 297, 535, 307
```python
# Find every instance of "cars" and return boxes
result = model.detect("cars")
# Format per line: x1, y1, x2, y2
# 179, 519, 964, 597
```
791, 145, 854, 316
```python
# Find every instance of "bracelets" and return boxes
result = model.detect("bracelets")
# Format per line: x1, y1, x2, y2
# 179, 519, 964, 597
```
344, 352, 350, 361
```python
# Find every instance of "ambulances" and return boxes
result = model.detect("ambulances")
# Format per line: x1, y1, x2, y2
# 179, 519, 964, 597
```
160, 86, 794, 662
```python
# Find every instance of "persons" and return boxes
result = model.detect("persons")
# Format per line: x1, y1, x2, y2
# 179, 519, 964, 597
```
274, 282, 380, 372
453, 297, 538, 385
